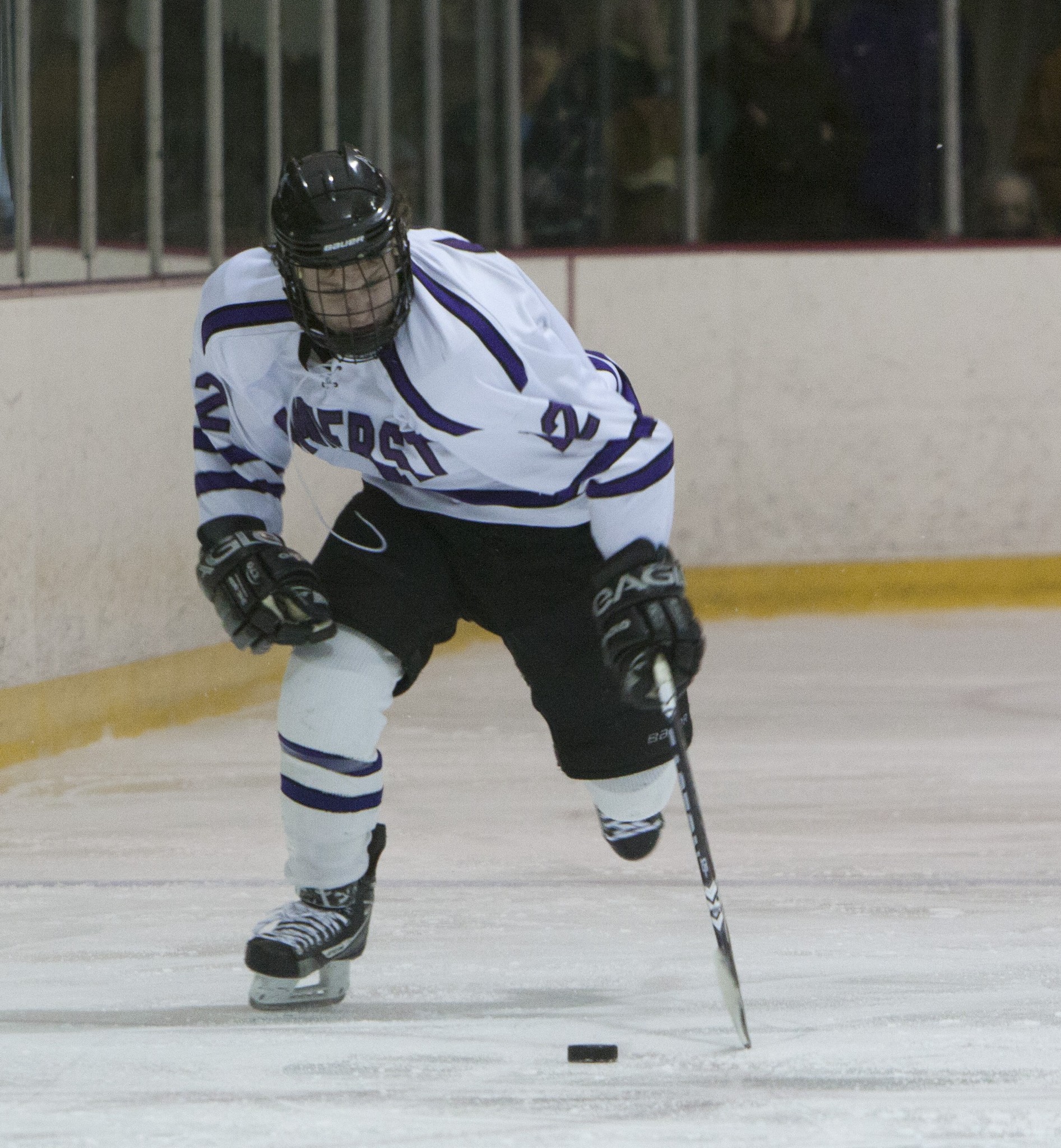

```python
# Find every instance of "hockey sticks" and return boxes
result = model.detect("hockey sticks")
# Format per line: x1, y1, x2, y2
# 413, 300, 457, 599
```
652, 652, 755, 1059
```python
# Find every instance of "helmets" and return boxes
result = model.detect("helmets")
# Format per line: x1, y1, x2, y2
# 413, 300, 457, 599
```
270, 141, 416, 363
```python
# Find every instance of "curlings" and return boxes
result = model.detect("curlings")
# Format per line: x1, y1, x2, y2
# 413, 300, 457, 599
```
566, 1043, 618, 1064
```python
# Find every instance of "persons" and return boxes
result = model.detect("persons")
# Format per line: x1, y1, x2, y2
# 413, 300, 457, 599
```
430, 0, 1061, 248
192, 147, 703, 1009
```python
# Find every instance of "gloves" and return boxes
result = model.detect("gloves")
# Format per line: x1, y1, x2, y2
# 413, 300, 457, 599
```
195, 516, 338, 655
590, 538, 707, 712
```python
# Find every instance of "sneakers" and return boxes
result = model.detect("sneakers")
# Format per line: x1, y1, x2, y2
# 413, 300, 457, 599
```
591, 804, 667, 861
244, 821, 385, 1013
